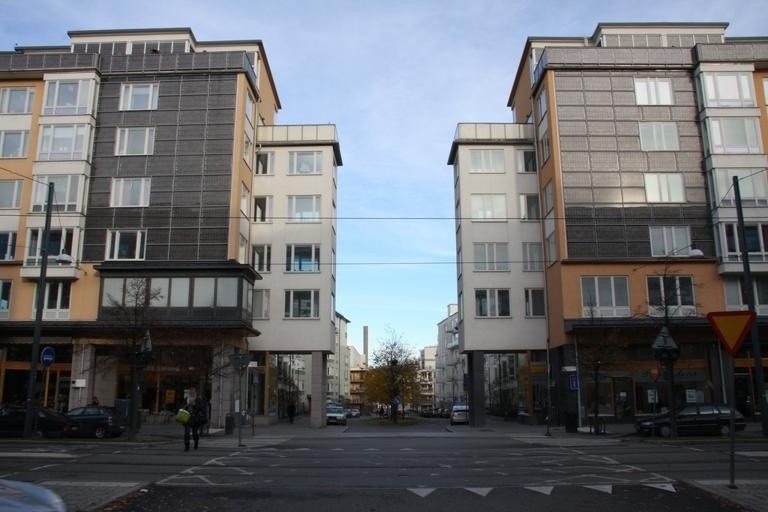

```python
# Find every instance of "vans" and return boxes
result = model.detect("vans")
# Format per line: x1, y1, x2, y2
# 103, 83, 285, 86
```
450, 405, 469, 425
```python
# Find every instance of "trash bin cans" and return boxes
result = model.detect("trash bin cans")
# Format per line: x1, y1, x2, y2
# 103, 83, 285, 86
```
565, 410, 578, 433
225, 413, 235, 435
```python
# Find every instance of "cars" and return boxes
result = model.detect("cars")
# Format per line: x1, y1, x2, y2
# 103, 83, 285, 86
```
422, 409, 448, 418
634, 405, 745, 437
66, 406, 127, 439
0, 406, 71, 438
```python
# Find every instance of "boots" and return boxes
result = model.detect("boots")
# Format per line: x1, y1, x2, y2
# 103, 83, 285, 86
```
183, 433, 190, 451
193, 434, 199, 448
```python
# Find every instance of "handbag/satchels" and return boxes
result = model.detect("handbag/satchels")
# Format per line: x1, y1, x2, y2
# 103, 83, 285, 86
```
177, 409, 191, 425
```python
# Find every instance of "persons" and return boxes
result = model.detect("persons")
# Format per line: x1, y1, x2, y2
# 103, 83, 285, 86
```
88, 395, 100, 406
377, 406, 384, 417
285, 397, 297, 423
180, 386, 207, 451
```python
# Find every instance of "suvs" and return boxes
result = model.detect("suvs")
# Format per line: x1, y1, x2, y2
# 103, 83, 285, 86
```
325, 403, 360, 426
